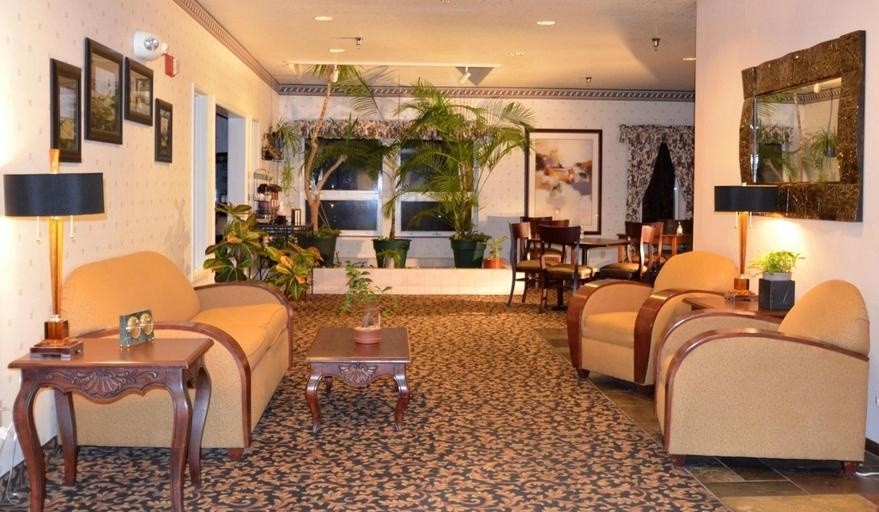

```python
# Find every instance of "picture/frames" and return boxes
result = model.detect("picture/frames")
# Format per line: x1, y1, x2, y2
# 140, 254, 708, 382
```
523, 129, 603, 235
49, 58, 82, 164
82, 36, 124, 145
155, 97, 174, 163
124, 57, 154, 126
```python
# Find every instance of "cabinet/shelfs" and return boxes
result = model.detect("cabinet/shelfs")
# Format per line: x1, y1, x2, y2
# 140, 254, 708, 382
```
252, 168, 273, 224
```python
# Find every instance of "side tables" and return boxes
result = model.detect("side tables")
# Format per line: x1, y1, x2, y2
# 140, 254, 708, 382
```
681, 293, 790, 318
6, 337, 214, 512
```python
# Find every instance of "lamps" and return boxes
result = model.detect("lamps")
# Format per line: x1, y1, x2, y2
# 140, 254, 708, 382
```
457, 67, 470, 85
714, 182, 780, 301
3, 149, 106, 362
329, 63, 339, 82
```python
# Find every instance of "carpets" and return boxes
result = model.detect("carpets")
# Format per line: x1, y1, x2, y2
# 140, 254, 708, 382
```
2, 287, 732, 512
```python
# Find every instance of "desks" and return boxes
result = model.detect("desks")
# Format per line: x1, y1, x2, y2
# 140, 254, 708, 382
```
543, 237, 632, 293
615, 232, 692, 263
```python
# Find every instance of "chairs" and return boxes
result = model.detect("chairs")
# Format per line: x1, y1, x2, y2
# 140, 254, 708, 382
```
600, 221, 655, 281
539, 220, 569, 263
624, 221, 650, 263
507, 223, 563, 310
519, 216, 565, 292
644, 221, 664, 280
539, 223, 592, 309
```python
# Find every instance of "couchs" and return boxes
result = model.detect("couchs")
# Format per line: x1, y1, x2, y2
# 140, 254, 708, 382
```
56, 251, 293, 461
654, 281, 870, 481
567, 252, 742, 394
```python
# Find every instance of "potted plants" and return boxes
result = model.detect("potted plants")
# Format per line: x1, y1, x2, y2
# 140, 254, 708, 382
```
340, 260, 392, 346
291, 63, 487, 268
375, 246, 404, 269
747, 250, 807, 281
483, 236, 508, 266
274, 112, 359, 264
203, 200, 268, 283
756, 117, 793, 184
379, 77, 536, 268
808, 125, 842, 171
